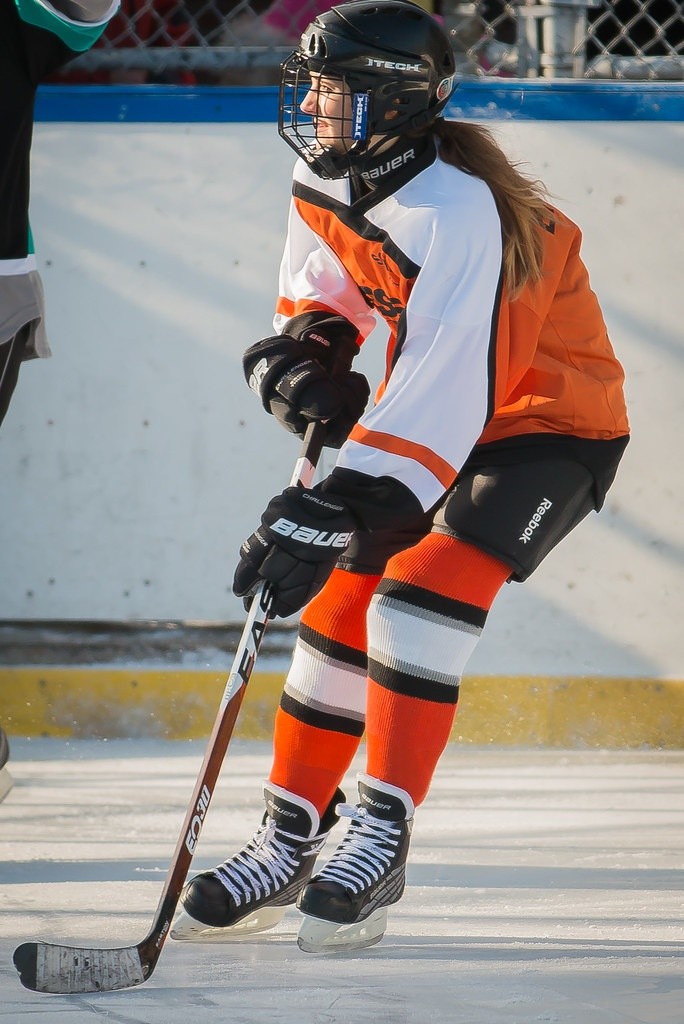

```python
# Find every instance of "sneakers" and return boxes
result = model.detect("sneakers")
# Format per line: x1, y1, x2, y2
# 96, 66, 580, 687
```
298, 772, 413, 954
170, 781, 346, 940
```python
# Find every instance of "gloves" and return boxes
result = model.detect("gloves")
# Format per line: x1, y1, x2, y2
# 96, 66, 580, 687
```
233, 496, 355, 619
243, 315, 370, 448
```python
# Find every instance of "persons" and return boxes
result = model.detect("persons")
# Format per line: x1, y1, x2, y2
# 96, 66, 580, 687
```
168, 1, 633, 954
44, 1, 519, 84
0, 0, 122, 805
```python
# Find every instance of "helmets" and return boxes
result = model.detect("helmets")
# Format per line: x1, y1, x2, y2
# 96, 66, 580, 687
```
278, 0, 456, 180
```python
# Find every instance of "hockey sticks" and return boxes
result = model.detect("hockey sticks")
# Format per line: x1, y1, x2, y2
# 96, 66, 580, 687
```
10, 420, 327, 995
